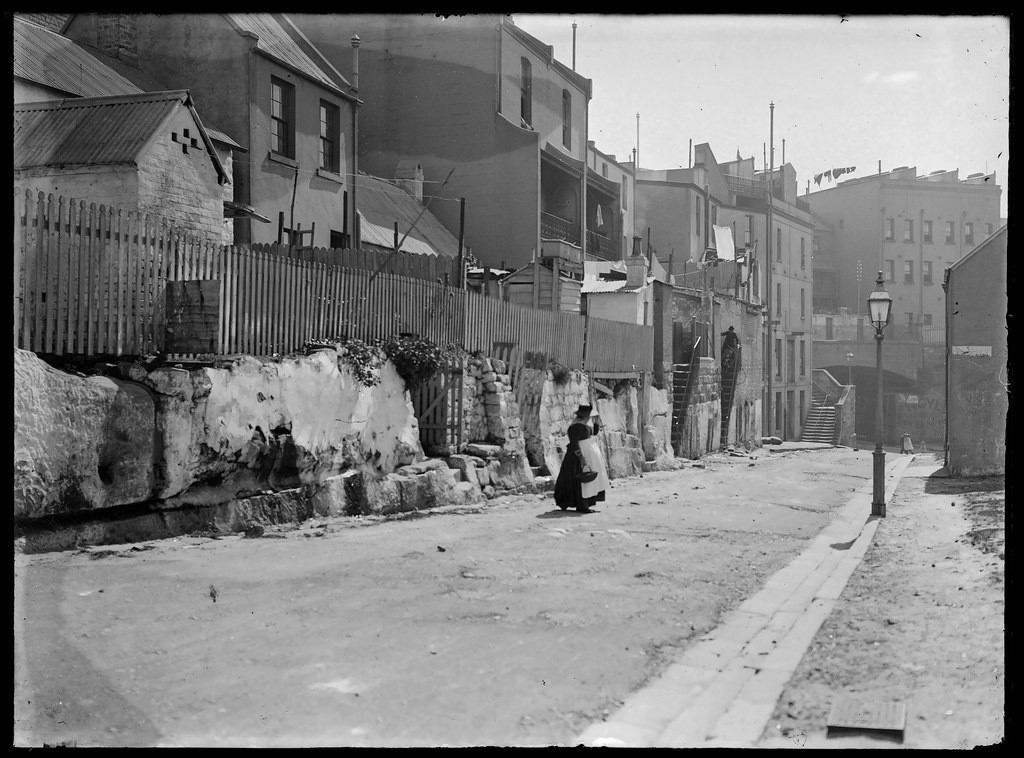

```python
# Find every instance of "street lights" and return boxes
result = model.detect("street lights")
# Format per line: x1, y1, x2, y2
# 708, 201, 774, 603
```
865, 269, 893, 522
846, 346, 855, 384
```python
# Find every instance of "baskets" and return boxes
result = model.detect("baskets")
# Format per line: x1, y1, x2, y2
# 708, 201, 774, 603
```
576, 464, 597, 484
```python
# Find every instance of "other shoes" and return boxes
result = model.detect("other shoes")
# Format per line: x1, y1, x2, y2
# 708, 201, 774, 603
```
560, 506, 568, 510
576, 507, 594, 513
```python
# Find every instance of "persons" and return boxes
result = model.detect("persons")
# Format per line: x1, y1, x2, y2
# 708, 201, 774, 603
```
554, 404, 608, 513
720, 326, 742, 353
900, 432, 913, 454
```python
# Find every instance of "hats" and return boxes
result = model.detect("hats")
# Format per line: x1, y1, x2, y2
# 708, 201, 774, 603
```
573, 404, 599, 417
